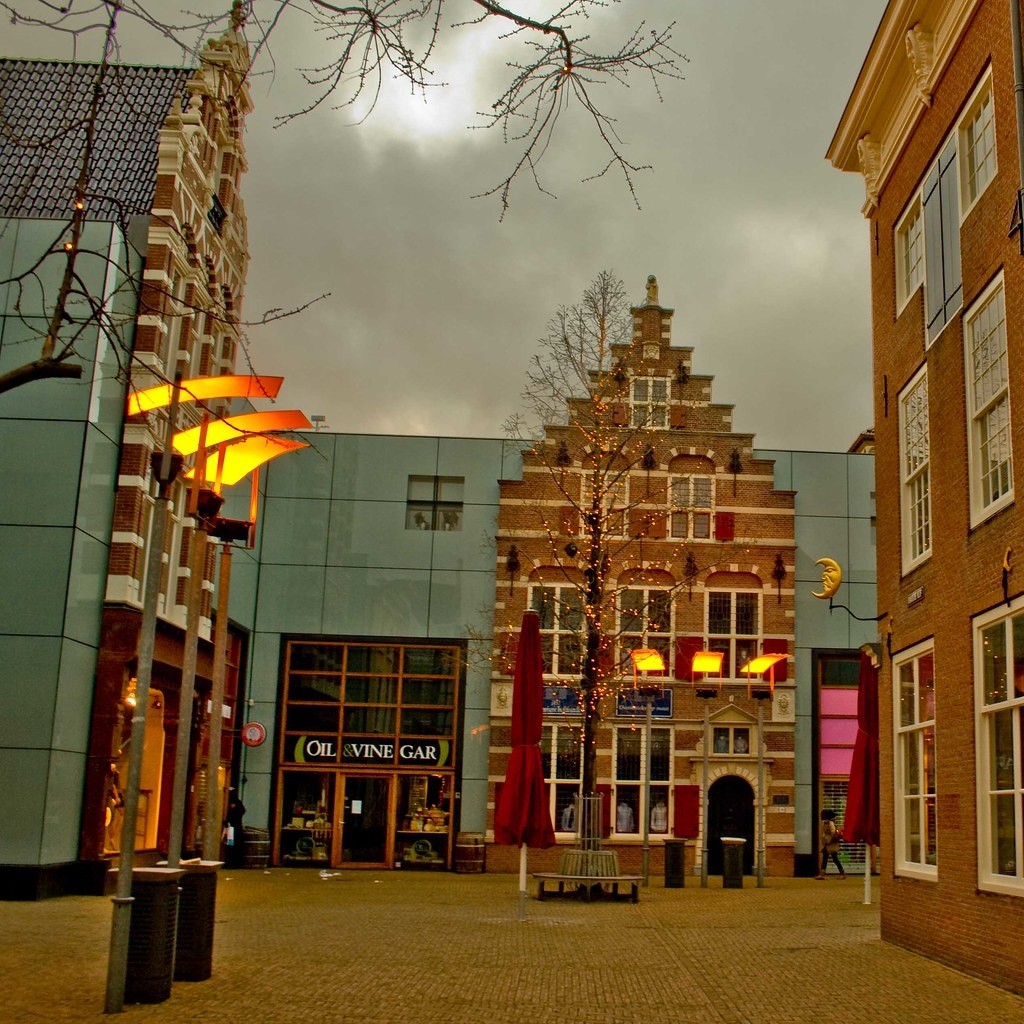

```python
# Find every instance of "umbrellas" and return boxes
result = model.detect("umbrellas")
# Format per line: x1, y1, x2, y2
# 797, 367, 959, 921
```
493, 605, 556, 920
841, 649, 880, 903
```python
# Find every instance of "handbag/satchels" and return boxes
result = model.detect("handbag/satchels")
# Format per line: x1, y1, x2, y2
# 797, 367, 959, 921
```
222, 822, 235, 845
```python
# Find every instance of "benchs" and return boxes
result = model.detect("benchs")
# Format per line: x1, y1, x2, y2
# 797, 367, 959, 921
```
531, 872, 647, 902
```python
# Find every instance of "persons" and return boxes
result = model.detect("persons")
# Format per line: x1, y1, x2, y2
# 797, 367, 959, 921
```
815, 809, 846, 879
104, 763, 125, 851
651, 802, 668, 832
221, 797, 246, 870
562, 805, 576, 831
617, 803, 634, 832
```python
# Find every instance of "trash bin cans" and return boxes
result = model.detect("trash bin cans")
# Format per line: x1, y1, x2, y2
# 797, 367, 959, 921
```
108, 865, 188, 1006
452, 831, 486, 874
241, 824, 271, 869
661, 838, 688, 890
720, 836, 747, 889
156, 858, 225, 984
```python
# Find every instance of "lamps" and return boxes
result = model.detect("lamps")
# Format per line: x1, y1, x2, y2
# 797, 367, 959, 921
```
752, 690, 771, 699
695, 688, 718, 699
212, 516, 252, 553
186, 487, 227, 531
639, 686, 660, 697
147, 451, 182, 501
